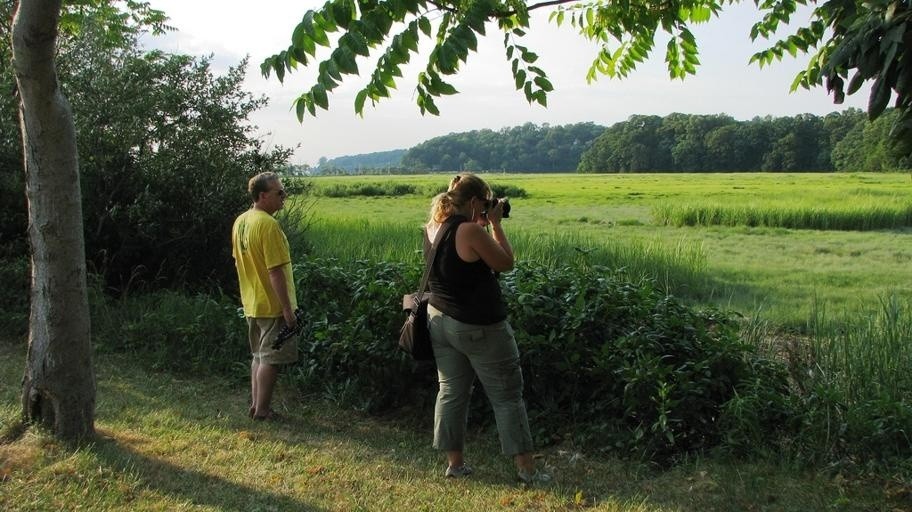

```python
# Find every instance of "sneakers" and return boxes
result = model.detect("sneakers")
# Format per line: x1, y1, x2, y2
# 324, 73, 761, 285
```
445, 467, 474, 478
517, 470, 551, 483
247, 407, 284, 422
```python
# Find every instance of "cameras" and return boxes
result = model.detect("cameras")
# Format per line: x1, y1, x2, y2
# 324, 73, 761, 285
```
481, 199, 510, 218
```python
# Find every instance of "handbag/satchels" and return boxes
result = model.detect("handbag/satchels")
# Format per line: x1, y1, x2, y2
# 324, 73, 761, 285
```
399, 291, 434, 361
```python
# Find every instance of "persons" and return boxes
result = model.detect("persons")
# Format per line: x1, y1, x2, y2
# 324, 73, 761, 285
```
232, 171, 299, 425
423, 173, 560, 488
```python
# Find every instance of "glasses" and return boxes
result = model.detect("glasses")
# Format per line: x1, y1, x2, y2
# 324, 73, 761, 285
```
266, 189, 284, 195
478, 196, 492, 208
